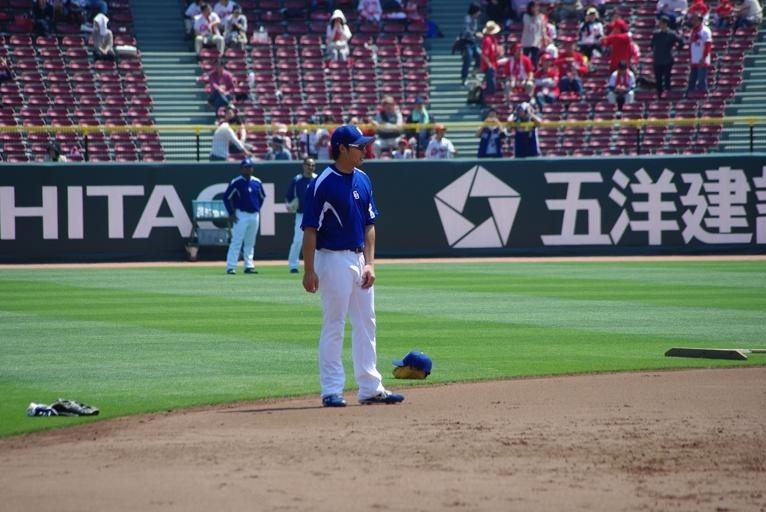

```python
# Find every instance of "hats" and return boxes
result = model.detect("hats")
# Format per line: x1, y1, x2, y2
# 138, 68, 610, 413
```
482, 21, 501, 35
392, 352, 431, 372
240, 159, 253, 167
331, 125, 375, 145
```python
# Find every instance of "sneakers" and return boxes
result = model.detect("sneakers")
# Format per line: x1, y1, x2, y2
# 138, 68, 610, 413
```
323, 395, 346, 406
360, 390, 404, 405
228, 268, 258, 274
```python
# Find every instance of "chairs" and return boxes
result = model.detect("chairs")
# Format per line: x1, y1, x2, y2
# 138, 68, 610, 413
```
483, 0, 758, 156
183, 1, 435, 162
0, 1, 164, 163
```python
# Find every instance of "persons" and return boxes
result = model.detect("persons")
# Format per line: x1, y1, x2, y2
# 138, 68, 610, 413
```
284, 159, 319, 273
300, 123, 405, 408
221, 158, 266, 275
31, 1, 115, 61
186, 0, 764, 159
43, 142, 67, 162
